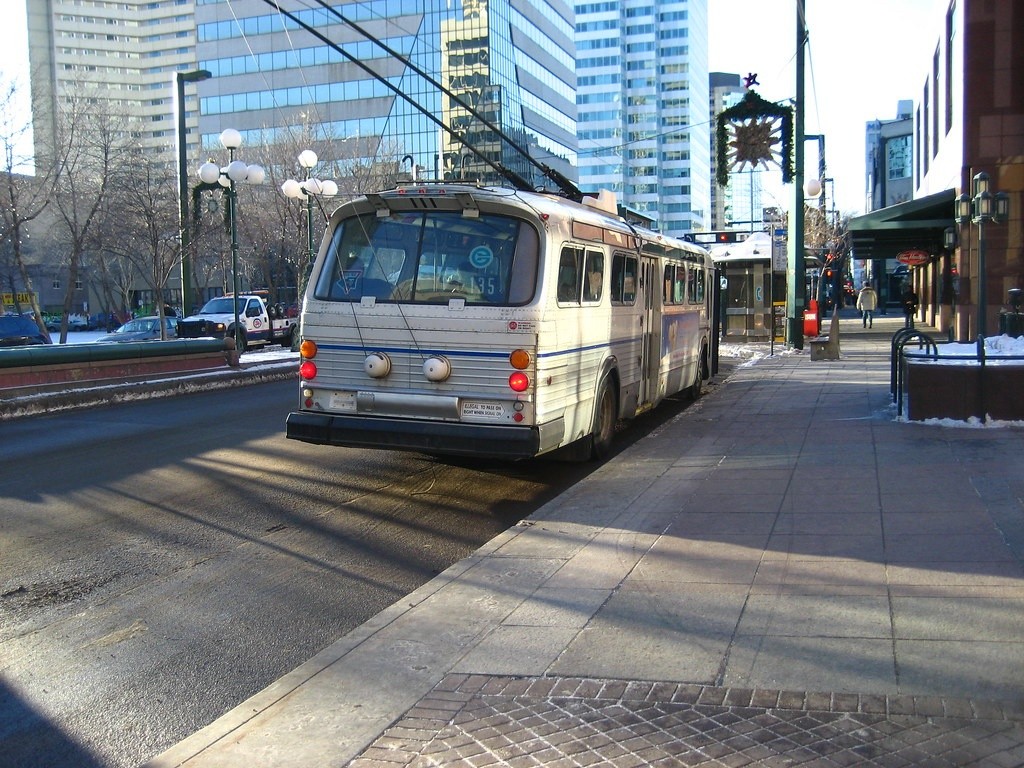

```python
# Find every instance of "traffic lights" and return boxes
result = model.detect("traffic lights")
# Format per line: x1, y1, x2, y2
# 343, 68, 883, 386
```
716, 233, 736, 242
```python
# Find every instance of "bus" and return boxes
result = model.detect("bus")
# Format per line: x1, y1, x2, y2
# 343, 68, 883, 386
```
258, 0, 727, 471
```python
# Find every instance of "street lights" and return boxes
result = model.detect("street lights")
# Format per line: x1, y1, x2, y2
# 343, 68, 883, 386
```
280, 148, 339, 272
956, 169, 1010, 425
768, 223, 776, 357
197, 127, 264, 372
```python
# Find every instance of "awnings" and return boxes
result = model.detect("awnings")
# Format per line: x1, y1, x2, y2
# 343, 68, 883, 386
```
848, 188, 957, 260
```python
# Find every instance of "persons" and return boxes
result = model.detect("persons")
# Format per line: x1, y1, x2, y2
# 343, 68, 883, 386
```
901, 285, 919, 335
856, 281, 878, 330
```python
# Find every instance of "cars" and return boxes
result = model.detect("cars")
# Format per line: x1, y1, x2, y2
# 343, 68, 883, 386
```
0, 310, 97, 348
95, 314, 185, 342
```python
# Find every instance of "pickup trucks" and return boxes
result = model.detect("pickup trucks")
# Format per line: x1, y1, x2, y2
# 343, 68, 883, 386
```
177, 292, 300, 356
